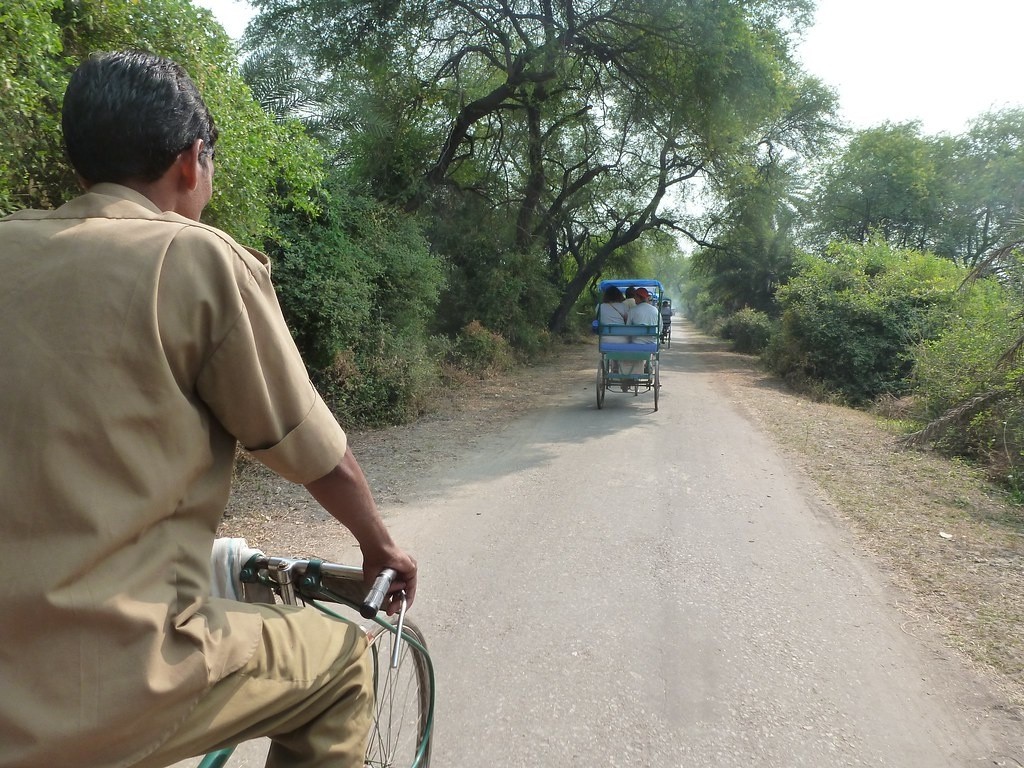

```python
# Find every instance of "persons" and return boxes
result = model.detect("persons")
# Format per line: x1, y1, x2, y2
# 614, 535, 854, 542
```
1, 48, 418, 768
626, 288, 664, 373
595, 286, 628, 374
622, 286, 637, 308
661, 301, 672, 335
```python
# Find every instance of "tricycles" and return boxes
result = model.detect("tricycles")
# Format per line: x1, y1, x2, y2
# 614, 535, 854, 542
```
592, 279, 664, 411
652, 296, 673, 349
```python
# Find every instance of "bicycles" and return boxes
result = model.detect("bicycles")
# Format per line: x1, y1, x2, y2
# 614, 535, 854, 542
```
196, 553, 435, 768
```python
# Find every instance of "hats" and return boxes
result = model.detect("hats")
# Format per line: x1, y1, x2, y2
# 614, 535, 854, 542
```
625, 287, 636, 295
635, 287, 649, 298
